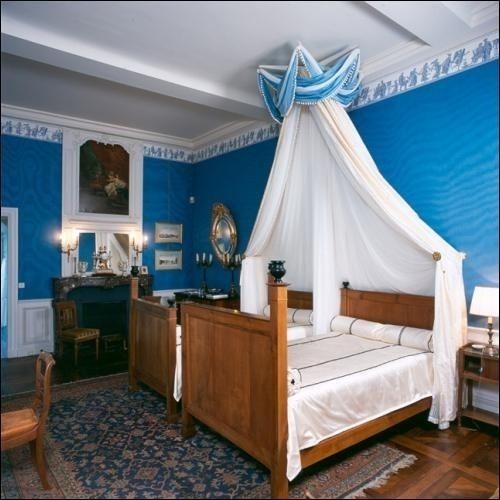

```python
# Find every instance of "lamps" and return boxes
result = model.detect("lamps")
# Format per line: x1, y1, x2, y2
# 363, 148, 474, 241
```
469, 286, 500, 356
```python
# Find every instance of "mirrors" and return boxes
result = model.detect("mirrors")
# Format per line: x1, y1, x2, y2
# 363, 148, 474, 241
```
209, 200, 239, 269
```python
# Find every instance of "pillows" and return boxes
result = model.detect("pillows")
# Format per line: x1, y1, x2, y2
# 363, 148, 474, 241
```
262, 300, 316, 327
332, 315, 434, 353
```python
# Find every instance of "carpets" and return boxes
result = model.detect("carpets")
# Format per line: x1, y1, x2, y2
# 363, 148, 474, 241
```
0, 372, 419, 500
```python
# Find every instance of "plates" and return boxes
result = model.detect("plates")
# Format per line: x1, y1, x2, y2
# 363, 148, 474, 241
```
471, 345, 486, 350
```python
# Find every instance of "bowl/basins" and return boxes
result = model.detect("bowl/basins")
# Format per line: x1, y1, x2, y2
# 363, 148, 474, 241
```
210, 288, 222, 295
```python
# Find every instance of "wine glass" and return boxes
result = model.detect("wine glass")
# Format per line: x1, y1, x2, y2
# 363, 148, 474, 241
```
78, 262, 87, 278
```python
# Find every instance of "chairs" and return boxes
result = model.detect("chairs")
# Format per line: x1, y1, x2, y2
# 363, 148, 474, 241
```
0, 351, 57, 491
55, 299, 102, 364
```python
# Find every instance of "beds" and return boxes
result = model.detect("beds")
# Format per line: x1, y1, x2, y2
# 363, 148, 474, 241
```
129, 268, 316, 425
182, 263, 435, 500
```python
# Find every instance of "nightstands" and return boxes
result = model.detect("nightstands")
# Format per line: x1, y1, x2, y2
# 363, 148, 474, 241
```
457, 342, 499, 439
